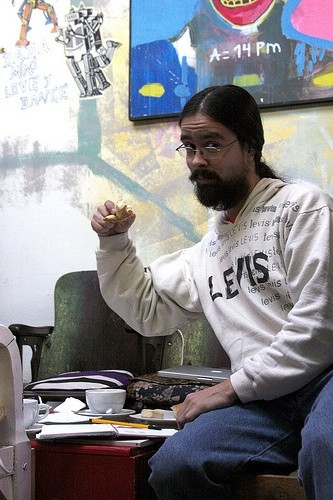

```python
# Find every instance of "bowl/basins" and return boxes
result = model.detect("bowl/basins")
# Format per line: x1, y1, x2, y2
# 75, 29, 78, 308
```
85, 388, 126, 413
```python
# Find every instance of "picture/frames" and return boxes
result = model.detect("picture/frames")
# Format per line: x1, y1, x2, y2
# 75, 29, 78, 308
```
128, 0, 333, 124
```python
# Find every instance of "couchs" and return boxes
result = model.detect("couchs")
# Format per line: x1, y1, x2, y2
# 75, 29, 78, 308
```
8, 269, 333, 500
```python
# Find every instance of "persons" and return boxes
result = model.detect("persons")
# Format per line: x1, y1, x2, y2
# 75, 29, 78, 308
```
90, 85, 333, 500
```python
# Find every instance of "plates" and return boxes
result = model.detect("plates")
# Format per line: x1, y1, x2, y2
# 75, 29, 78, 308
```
78, 409, 136, 419
129, 413, 177, 423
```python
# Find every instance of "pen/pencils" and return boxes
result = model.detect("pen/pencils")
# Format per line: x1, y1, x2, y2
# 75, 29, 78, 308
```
89, 418, 162, 431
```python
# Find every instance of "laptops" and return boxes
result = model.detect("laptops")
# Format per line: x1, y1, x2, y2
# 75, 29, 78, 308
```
157, 365, 233, 384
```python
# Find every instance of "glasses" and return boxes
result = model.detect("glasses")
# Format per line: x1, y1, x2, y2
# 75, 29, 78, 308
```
176, 139, 238, 159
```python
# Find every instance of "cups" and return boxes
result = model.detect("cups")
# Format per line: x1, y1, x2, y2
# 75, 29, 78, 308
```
23, 399, 49, 429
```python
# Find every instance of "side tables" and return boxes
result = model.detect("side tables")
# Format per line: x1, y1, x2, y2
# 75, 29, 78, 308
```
27, 440, 158, 500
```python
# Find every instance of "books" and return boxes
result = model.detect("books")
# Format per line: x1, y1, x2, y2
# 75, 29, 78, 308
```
37, 423, 180, 440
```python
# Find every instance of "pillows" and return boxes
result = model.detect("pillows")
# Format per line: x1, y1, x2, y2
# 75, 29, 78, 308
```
23, 369, 135, 398
126, 376, 210, 411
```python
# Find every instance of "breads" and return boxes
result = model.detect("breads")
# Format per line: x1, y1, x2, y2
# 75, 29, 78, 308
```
141, 409, 164, 419
170, 403, 181, 417
